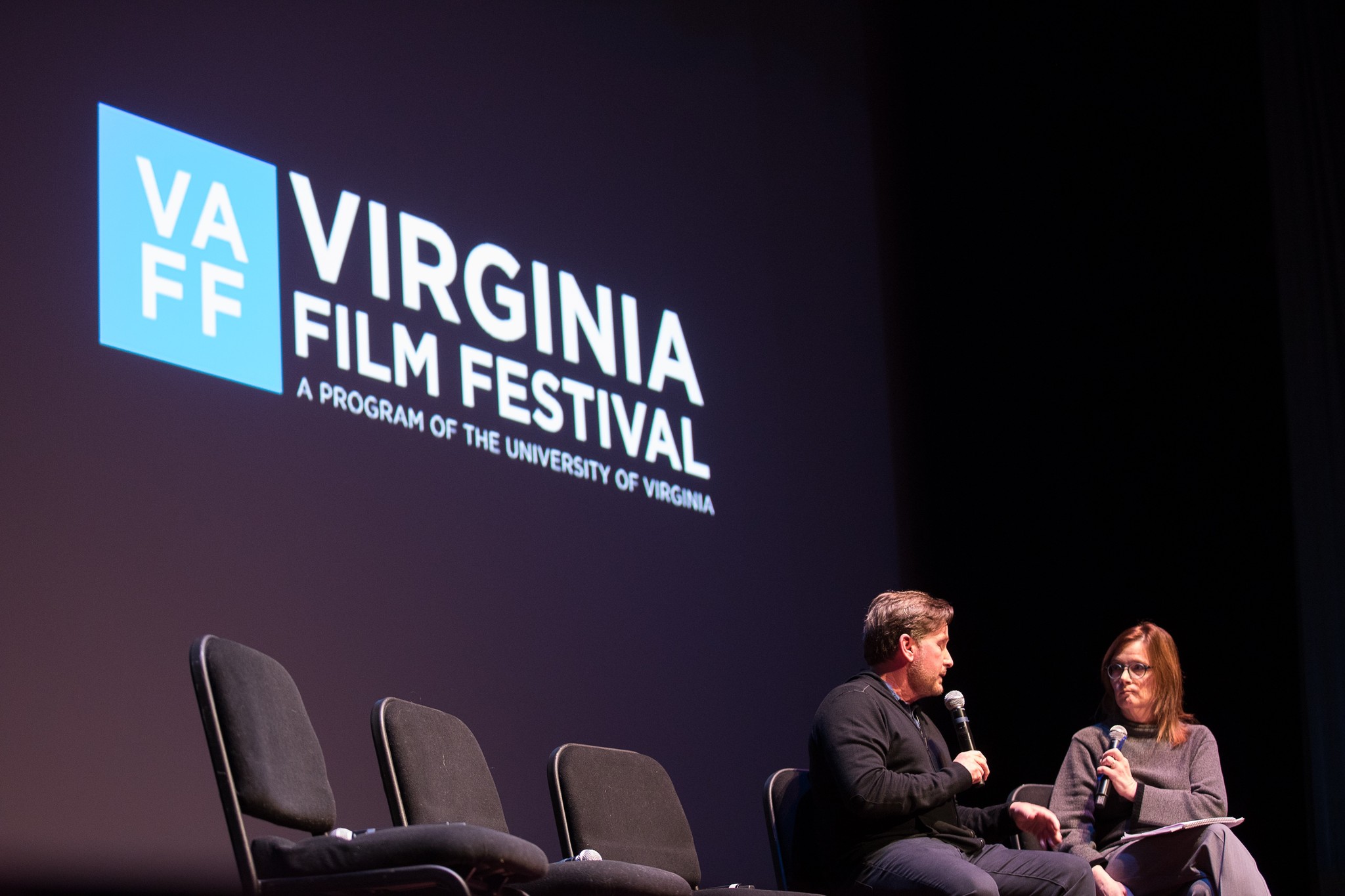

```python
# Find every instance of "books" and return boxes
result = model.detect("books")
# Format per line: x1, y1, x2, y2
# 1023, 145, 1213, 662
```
1121, 816, 1245, 838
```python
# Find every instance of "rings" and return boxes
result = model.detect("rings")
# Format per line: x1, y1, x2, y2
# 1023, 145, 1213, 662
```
1110, 760, 1116, 767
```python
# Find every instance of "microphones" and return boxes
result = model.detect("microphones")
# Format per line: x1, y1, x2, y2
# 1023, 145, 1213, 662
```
944, 691, 986, 786
329, 827, 384, 841
552, 850, 603, 863
1095, 724, 1127, 808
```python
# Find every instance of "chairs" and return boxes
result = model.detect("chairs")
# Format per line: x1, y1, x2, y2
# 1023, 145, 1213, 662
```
369, 697, 692, 896
1006, 784, 1054, 851
544, 742, 826, 896
763, 768, 810, 891
189, 634, 549, 896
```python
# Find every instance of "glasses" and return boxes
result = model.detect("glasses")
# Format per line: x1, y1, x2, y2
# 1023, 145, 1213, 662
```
1106, 662, 1152, 680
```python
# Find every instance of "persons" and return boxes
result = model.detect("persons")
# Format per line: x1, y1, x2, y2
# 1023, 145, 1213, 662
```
812, 590, 1098, 896
1047, 622, 1276, 896
